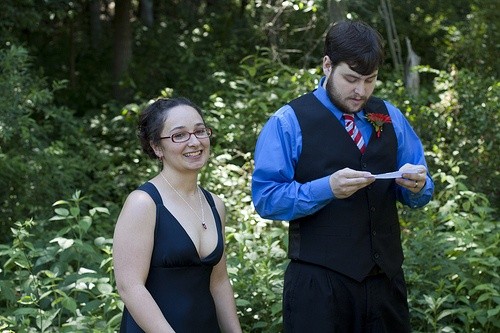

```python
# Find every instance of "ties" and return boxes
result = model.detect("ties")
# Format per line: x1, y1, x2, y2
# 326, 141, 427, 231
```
342, 114, 366, 155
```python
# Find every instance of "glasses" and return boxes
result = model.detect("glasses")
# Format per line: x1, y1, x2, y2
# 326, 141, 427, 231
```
157, 128, 212, 143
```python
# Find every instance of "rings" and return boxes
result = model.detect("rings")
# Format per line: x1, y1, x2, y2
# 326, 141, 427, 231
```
414, 181, 418, 188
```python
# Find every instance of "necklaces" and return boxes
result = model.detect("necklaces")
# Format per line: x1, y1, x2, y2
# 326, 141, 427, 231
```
160, 172, 208, 230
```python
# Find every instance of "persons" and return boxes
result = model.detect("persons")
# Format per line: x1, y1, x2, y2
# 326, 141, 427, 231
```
113, 98, 242, 333
251, 21, 435, 333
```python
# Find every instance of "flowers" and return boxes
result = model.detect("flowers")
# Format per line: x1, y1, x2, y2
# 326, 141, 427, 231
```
365, 112, 392, 138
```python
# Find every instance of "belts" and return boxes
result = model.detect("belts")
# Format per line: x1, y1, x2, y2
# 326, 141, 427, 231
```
367, 267, 384, 276
